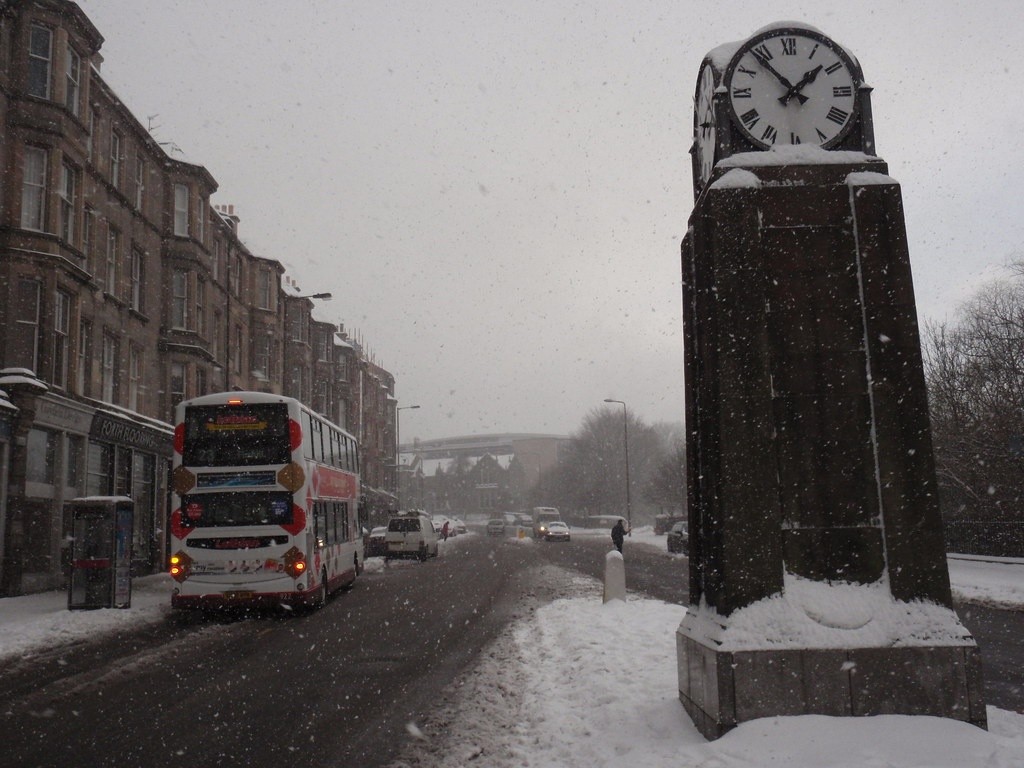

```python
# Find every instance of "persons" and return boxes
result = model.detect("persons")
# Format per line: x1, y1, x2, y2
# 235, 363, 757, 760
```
442, 521, 449, 542
611, 520, 627, 555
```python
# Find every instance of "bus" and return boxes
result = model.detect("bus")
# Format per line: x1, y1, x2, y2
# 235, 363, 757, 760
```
167, 390, 368, 618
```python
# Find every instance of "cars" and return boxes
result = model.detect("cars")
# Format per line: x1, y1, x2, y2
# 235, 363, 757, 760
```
430, 514, 467, 537
503, 514, 519, 527
487, 518, 506, 537
545, 521, 571, 542
666, 520, 690, 556
367, 525, 387, 557
518, 514, 535, 527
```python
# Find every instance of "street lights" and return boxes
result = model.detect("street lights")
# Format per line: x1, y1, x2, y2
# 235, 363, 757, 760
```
604, 398, 632, 538
395, 405, 420, 511
282, 290, 334, 396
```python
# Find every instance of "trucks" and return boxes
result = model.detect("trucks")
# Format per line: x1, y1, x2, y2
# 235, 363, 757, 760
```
533, 506, 561, 537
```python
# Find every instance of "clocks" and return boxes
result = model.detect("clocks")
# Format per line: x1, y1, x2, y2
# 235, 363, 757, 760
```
722, 26, 861, 151
691, 52, 720, 191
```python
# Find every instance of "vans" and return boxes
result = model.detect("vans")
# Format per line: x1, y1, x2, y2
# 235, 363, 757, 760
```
383, 515, 442, 562
